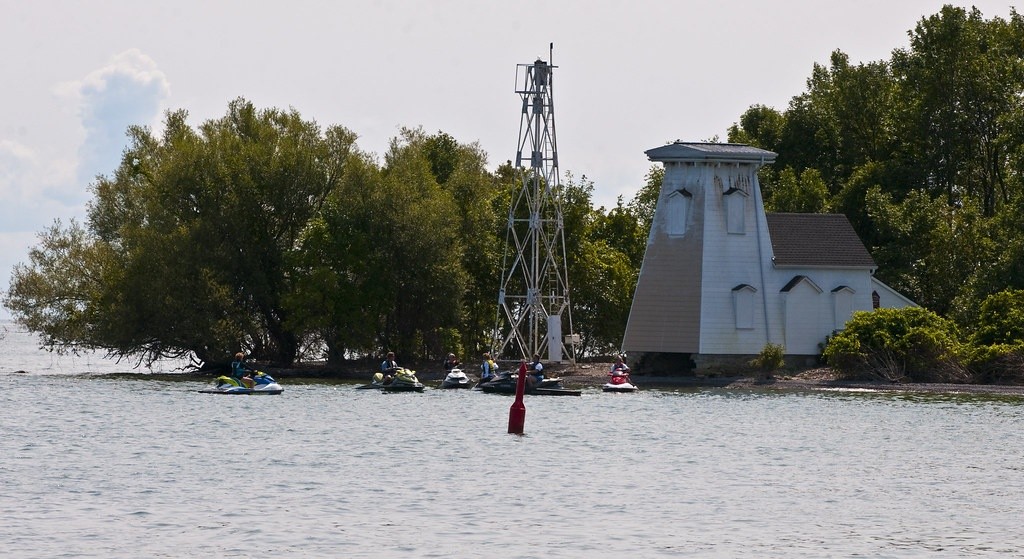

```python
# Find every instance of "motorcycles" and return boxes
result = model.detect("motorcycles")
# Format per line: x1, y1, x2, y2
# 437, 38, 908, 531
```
602, 368, 638, 393
479, 369, 583, 397
357, 365, 425, 391
198, 369, 286, 394
438, 367, 471, 390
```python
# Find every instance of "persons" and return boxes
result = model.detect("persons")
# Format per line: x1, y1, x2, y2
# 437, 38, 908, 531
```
609, 355, 630, 373
526, 354, 544, 387
443, 352, 459, 375
481, 353, 498, 383
381, 352, 398, 385
232, 353, 255, 388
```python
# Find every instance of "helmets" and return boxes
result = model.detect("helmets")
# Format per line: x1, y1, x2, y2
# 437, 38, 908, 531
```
236, 353, 243, 357
482, 353, 491, 360
